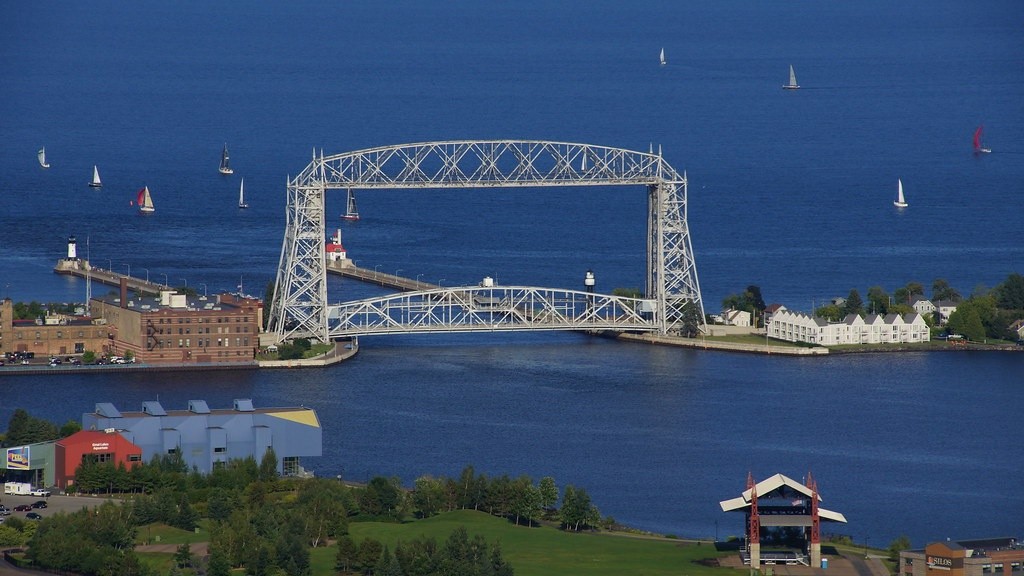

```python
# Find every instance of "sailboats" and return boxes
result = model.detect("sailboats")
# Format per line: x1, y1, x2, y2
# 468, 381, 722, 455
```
37, 147, 50, 168
782, 64, 801, 88
88, 165, 102, 186
660, 48, 667, 64
974, 124, 991, 153
342, 190, 359, 219
238, 178, 249, 208
137, 186, 155, 213
218, 142, 233, 173
893, 178, 909, 207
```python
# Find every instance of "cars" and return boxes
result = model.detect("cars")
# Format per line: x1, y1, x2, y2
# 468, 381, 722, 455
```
13, 505, 30, 511
0, 508, 10, 516
26, 513, 41, 519
31, 501, 47, 508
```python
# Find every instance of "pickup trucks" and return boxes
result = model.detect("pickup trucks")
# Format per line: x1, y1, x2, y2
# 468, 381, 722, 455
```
31, 489, 50, 496
